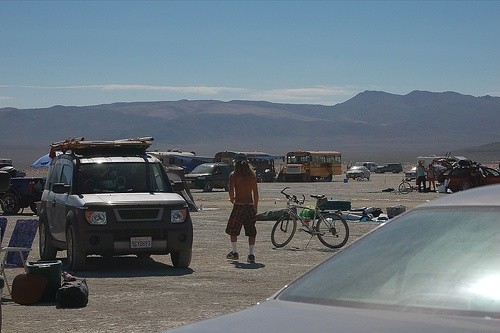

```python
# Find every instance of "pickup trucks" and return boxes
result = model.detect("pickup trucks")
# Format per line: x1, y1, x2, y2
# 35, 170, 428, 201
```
0, 159, 47, 215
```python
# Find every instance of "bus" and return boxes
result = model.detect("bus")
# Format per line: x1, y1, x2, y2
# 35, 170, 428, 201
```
146, 150, 276, 182
281, 150, 342, 181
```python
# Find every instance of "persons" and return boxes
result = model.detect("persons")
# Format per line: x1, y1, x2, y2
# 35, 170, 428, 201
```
225, 153, 258, 263
427, 164, 436, 192
416, 163, 427, 192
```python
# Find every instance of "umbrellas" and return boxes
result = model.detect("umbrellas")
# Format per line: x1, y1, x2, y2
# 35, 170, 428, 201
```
30, 151, 69, 168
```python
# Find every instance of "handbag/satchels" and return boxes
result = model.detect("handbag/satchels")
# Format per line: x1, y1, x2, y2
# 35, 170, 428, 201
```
57, 278, 89, 309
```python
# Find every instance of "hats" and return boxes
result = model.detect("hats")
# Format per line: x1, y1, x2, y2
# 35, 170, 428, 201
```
234, 153, 250, 164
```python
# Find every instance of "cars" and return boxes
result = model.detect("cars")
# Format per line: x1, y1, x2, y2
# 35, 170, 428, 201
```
406, 156, 500, 192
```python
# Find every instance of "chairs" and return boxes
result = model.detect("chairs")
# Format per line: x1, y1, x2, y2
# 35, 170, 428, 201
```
0, 220, 38, 296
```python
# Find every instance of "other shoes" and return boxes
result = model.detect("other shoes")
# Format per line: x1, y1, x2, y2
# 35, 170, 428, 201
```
226, 251, 239, 261
247, 255, 256, 264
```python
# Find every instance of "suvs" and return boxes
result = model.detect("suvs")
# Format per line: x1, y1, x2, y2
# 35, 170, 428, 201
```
346, 162, 403, 181
185, 162, 233, 192
39, 137, 193, 270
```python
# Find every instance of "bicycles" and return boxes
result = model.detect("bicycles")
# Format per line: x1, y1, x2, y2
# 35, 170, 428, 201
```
271, 186, 349, 249
399, 177, 424, 194
